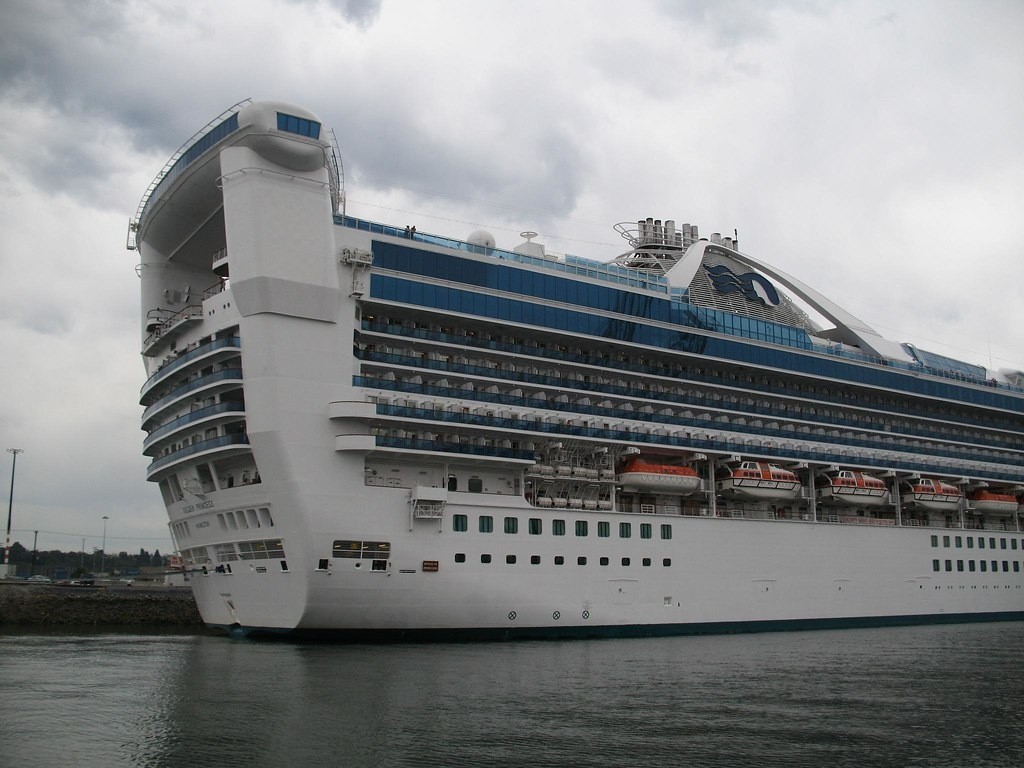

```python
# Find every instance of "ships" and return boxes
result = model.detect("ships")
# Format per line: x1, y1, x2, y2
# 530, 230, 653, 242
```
123, 92, 1024, 645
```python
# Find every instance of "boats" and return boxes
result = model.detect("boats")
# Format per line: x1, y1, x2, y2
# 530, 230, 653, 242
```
616, 458, 702, 498
818, 470, 890, 509
901, 477, 965, 513
716, 461, 802, 504
966, 487, 1018, 519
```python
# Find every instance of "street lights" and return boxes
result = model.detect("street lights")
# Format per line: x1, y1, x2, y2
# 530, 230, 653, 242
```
100, 515, 110, 578
3, 447, 25, 564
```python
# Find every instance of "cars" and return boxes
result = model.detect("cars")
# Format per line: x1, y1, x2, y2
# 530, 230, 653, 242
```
28, 574, 52, 583
78, 572, 95, 585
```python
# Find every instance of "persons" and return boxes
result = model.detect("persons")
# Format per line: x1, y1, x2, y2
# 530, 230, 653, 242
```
565, 420, 571, 434
365, 344, 374, 360
